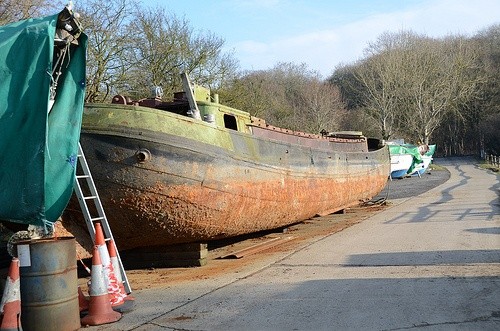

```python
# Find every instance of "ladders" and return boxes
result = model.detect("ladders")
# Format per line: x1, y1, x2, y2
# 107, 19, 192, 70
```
75, 139, 131, 295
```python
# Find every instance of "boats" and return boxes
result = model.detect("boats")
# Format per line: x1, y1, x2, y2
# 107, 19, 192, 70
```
385, 140, 436, 180
69, 73, 391, 244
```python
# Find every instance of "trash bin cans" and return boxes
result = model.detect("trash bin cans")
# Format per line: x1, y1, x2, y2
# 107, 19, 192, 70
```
12, 236, 81, 331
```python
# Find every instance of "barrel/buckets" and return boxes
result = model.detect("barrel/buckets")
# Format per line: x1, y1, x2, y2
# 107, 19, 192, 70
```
14, 235, 84, 331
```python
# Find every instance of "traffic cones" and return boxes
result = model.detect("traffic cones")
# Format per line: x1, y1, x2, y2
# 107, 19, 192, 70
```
73, 222, 138, 313
1, 257, 24, 331
77, 245, 121, 326
106, 240, 136, 300
